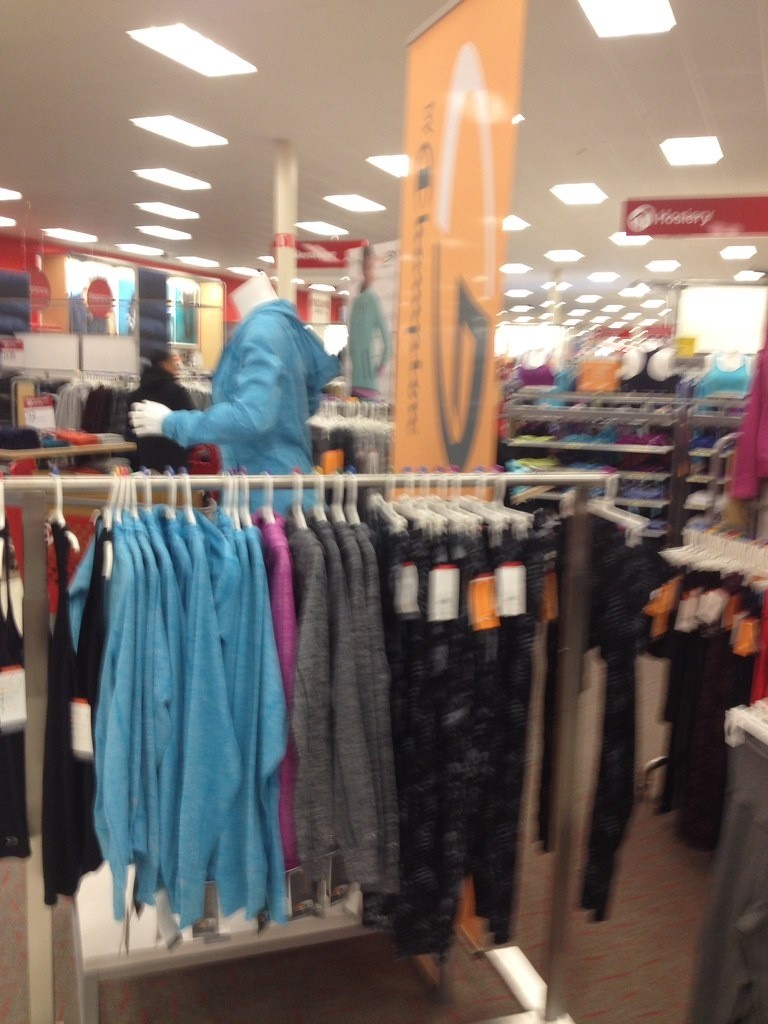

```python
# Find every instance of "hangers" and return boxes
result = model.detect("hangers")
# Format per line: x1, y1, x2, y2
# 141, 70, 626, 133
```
0, 367, 768, 747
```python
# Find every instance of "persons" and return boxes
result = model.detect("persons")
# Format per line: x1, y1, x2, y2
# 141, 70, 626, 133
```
516, 339, 753, 460
125, 349, 197, 477
127, 271, 344, 515
347, 246, 391, 400
